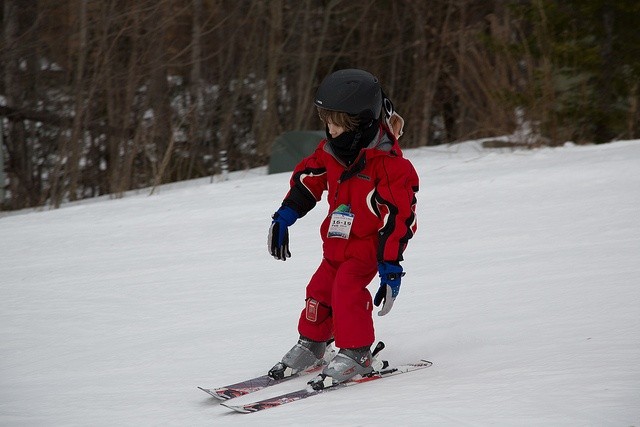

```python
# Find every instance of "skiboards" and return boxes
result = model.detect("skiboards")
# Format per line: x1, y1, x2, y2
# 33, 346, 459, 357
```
198, 360, 432, 413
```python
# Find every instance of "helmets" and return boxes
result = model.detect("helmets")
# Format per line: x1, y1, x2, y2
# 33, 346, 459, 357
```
314, 67, 382, 121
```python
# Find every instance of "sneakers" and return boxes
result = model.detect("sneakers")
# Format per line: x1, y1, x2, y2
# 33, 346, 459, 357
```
307, 346, 373, 392
267, 336, 324, 380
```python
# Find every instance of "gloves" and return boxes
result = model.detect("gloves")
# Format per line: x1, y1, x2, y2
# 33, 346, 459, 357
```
373, 259, 406, 317
267, 202, 299, 262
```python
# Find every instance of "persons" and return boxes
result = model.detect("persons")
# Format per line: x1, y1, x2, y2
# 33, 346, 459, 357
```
267, 68, 420, 389
